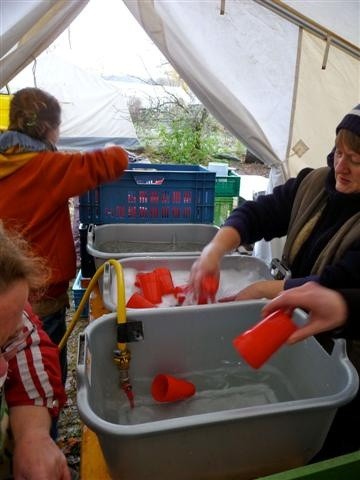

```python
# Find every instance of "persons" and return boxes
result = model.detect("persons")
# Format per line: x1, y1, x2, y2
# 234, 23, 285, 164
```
0, 218, 71, 480
261, 238, 360, 480
0, 87, 130, 440
189, 105, 360, 307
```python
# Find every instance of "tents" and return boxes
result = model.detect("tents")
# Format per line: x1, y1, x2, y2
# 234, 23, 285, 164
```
0, 0, 360, 272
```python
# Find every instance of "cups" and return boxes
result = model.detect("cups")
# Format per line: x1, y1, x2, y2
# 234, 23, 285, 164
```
152, 371, 196, 402
232, 309, 300, 368
112, 176, 189, 218
128, 270, 235, 310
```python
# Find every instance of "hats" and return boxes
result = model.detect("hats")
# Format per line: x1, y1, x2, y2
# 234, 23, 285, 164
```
336, 104, 360, 139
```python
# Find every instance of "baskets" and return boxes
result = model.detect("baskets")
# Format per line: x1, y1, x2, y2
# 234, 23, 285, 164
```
72, 268, 90, 317
80, 163, 216, 223
208, 170, 241, 227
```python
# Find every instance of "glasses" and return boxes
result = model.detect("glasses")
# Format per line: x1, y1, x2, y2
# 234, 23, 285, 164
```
0, 311, 36, 354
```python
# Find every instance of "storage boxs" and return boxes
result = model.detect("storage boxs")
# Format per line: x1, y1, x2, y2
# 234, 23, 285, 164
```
213, 197, 234, 227
75, 163, 214, 224
76, 297, 359, 480
72, 224, 96, 319
93, 254, 293, 321
205, 165, 241, 197
86, 223, 237, 294
